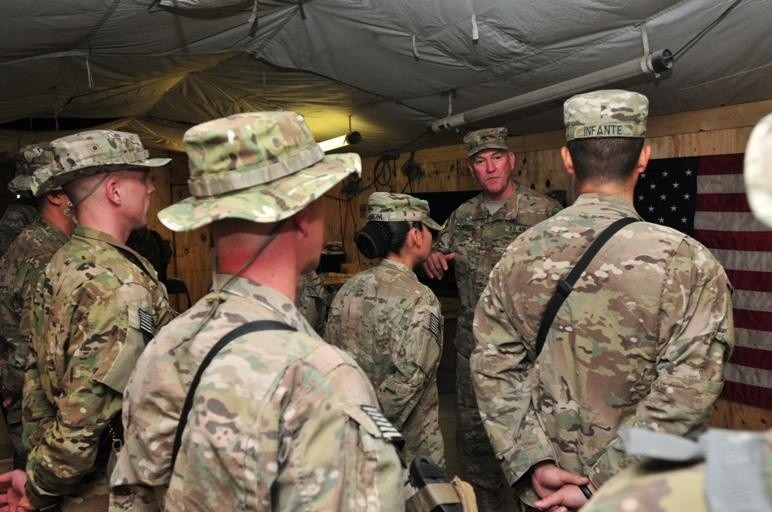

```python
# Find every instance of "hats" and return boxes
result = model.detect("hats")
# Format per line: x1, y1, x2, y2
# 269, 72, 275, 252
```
462, 127, 508, 160
562, 88, 650, 142
157, 110, 363, 235
30, 129, 173, 198
366, 191, 443, 231
8, 144, 63, 198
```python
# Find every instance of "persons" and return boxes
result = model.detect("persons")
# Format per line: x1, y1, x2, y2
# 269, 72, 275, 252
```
0, 130, 178, 512
325, 127, 563, 512
466, 89, 739, 511
579, 112, 772, 512
114, 110, 415, 512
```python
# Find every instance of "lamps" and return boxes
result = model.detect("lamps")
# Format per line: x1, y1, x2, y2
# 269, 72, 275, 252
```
425, 26, 673, 136
318, 113, 359, 154
